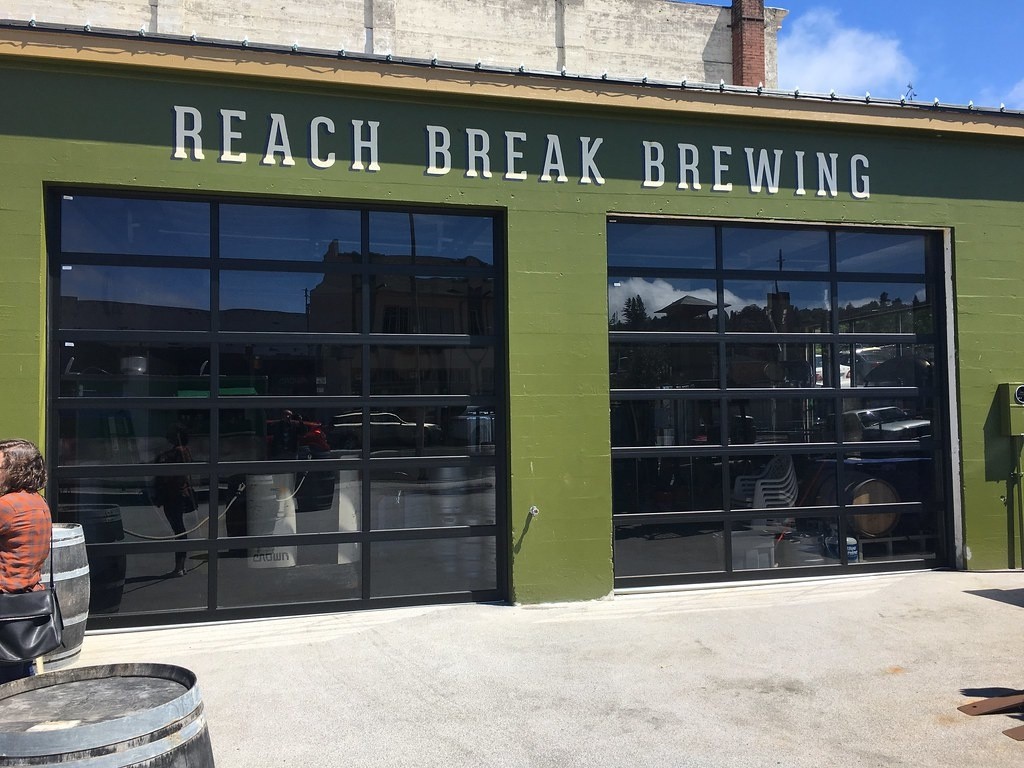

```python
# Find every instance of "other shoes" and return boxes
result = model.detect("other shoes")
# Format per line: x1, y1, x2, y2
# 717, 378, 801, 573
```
166, 568, 186, 577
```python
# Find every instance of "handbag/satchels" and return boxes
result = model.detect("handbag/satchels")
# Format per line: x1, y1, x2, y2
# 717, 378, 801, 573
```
0, 587, 66, 667
181, 486, 199, 513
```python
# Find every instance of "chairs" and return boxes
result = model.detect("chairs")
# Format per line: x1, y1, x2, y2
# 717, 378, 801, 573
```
733, 455, 797, 537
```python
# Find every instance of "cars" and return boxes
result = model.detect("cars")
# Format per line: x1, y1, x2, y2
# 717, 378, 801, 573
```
824, 403, 934, 457
852, 350, 896, 384
268, 418, 335, 452
328, 411, 446, 449
862, 356, 932, 389
813, 352, 852, 390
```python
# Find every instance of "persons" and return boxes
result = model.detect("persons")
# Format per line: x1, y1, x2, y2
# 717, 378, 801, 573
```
0, 438, 52, 685
271, 409, 305, 498
150, 421, 194, 576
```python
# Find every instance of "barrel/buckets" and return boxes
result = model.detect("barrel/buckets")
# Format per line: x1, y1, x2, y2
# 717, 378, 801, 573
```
823, 536, 859, 564
0, 663, 215, 768
810, 469, 901, 539
59, 505, 126, 616
27, 523, 90, 673
